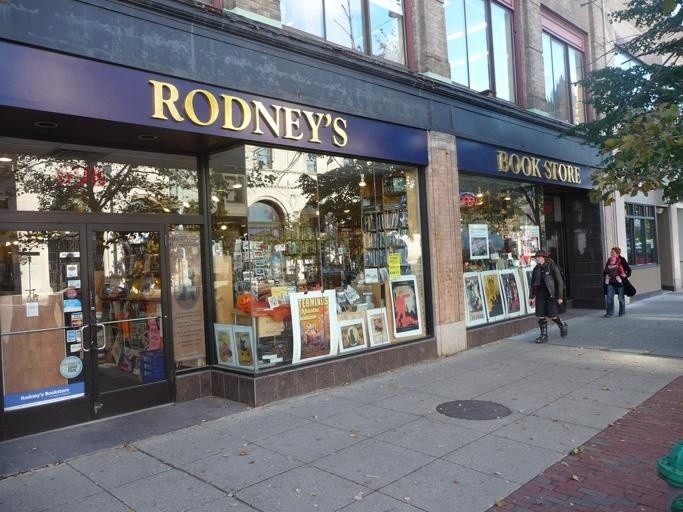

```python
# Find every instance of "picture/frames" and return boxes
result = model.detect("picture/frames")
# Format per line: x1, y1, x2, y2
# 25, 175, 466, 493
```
463, 267, 536, 330
232, 325, 258, 375
337, 318, 368, 353
366, 307, 392, 348
214, 324, 236, 368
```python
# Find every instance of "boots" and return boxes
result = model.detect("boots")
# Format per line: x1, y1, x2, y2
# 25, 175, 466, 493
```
535, 321, 547, 343
552, 315, 567, 338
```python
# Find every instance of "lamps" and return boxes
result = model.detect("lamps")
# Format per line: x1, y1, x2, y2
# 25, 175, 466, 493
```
358, 160, 367, 187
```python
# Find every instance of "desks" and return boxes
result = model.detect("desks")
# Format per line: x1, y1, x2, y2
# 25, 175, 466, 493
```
231, 308, 287, 357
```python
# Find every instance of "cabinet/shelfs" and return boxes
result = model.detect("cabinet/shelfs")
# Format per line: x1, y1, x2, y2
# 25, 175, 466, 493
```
357, 176, 420, 276
98, 244, 161, 375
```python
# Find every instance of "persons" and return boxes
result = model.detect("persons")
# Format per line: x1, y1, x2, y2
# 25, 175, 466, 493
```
529, 250, 568, 343
604, 256, 627, 285
509, 275, 519, 312
602, 248, 637, 319
395, 290, 411, 328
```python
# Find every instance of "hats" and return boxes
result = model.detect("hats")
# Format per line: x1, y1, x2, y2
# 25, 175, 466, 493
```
531, 251, 550, 258
609, 255, 616, 261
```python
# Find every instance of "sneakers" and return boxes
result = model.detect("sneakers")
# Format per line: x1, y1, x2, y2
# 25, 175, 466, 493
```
604, 314, 614, 318
